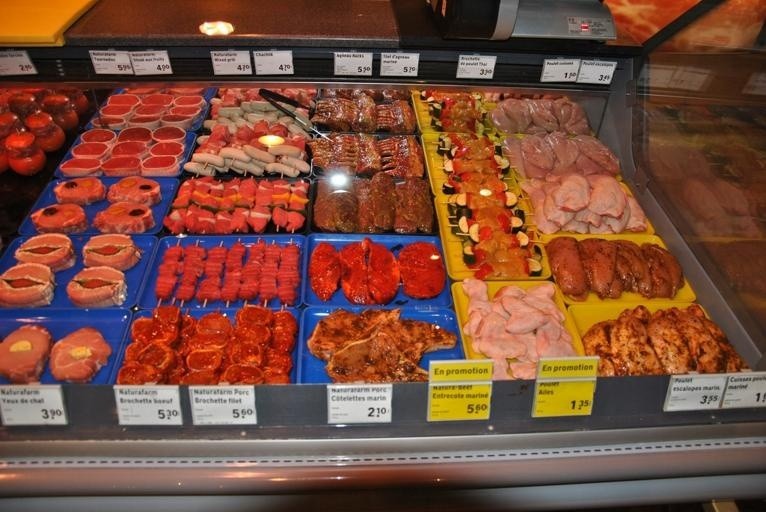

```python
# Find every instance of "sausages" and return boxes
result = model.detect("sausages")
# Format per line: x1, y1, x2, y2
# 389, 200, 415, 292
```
183, 98, 310, 177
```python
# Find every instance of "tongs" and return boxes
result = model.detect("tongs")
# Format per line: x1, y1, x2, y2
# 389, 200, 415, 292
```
256, 87, 338, 145
